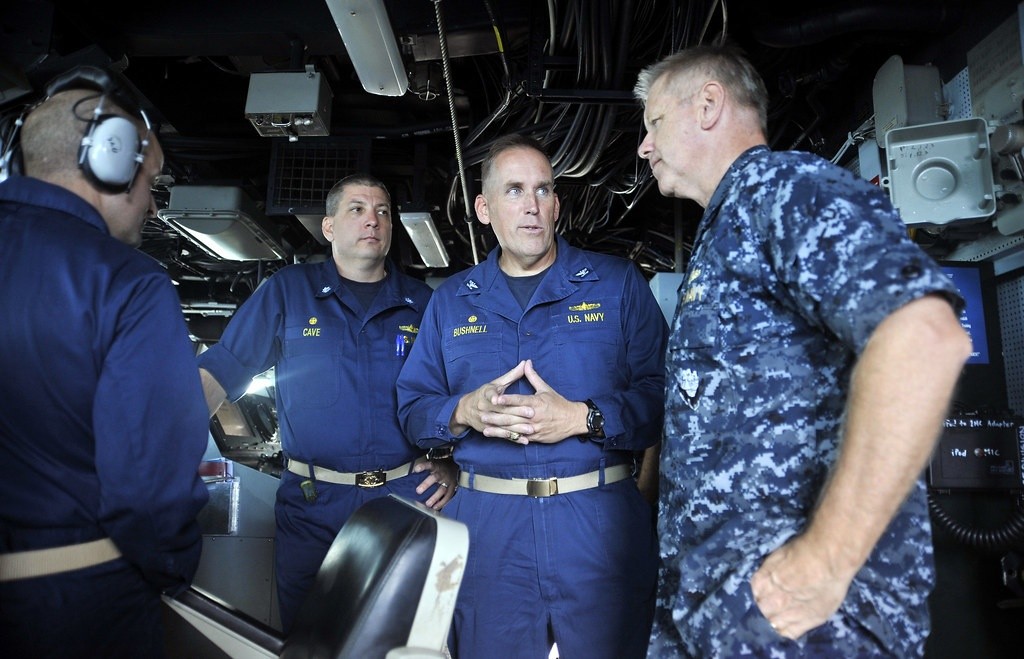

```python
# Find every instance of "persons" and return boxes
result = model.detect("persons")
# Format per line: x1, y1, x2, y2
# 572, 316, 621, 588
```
197, 173, 459, 636
634, 50, 973, 659
1, 91, 209, 659
394, 134, 671, 659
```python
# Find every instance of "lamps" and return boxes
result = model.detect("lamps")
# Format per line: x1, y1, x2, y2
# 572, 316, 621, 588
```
327, 0, 408, 97
289, 207, 331, 246
399, 212, 450, 267
157, 185, 288, 261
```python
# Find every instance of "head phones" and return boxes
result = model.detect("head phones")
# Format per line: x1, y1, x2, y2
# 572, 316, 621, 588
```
0, 66, 151, 195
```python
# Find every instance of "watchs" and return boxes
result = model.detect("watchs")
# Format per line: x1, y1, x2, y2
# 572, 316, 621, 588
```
580, 398, 605, 443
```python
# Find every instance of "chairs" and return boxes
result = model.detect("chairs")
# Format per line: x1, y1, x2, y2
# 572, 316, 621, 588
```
160, 493, 470, 659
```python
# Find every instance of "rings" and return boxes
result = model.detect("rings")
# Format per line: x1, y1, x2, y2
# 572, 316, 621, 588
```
440, 482, 448, 488
510, 432, 519, 440
